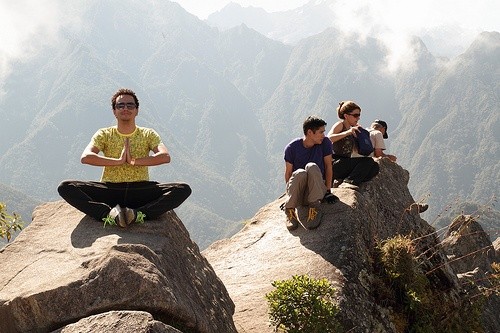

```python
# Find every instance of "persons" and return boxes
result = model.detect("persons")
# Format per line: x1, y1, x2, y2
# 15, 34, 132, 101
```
283, 115, 339, 230
57, 89, 192, 230
327, 100, 379, 190
351, 120, 398, 161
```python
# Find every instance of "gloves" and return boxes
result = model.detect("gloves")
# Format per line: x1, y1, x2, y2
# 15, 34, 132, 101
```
322, 189, 339, 204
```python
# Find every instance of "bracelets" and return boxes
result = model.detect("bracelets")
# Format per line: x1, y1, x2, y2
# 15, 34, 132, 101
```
130, 158, 135, 165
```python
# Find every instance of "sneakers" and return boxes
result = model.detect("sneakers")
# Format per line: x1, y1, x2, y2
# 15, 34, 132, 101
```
102, 203, 126, 229
124, 207, 146, 225
285, 208, 298, 230
306, 205, 324, 229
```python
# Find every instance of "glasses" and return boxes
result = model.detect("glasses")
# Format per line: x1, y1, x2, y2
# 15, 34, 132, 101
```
348, 113, 360, 118
115, 103, 137, 109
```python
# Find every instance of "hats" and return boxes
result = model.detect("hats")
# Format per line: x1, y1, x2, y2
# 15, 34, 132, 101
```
375, 120, 388, 139
351, 126, 374, 156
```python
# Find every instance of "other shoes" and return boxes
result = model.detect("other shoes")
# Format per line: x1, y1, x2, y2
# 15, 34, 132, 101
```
419, 204, 428, 213
